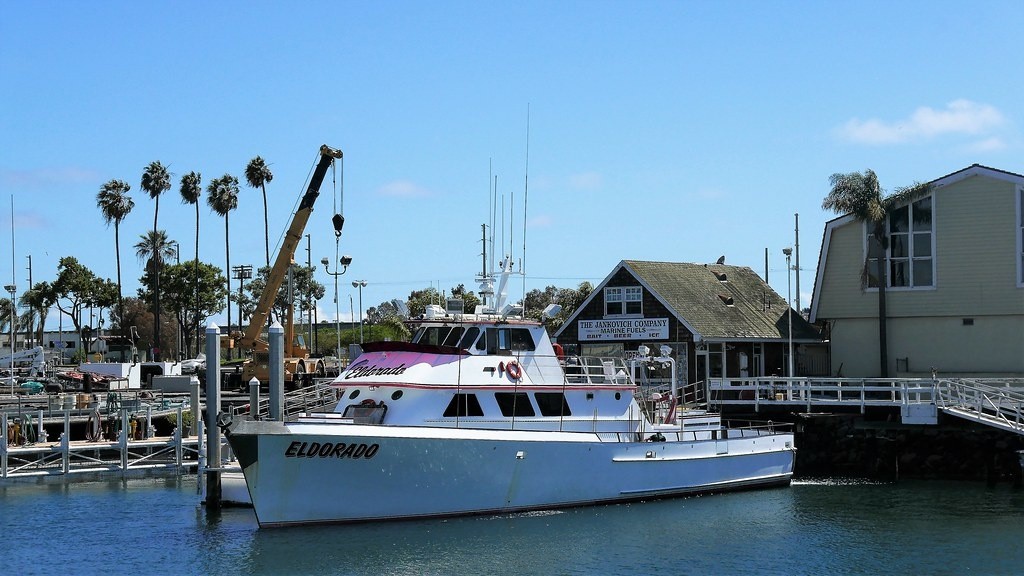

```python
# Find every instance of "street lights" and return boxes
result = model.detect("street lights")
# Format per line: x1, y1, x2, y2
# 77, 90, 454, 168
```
232, 265, 253, 359
353, 279, 367, 345
4, 285, 16, 400
783, 248, 793, 377
321, 256, 352, 376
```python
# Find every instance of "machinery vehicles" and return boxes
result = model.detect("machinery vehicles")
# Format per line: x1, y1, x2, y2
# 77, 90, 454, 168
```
220, 144, 346, 393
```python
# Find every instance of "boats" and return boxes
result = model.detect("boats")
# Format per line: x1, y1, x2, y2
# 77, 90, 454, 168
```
217, 104, 797, 528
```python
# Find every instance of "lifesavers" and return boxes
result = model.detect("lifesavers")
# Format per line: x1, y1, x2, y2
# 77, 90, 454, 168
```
552, 342, 564, 367
506, 360, 523, 380
93, 353, 103, 363
